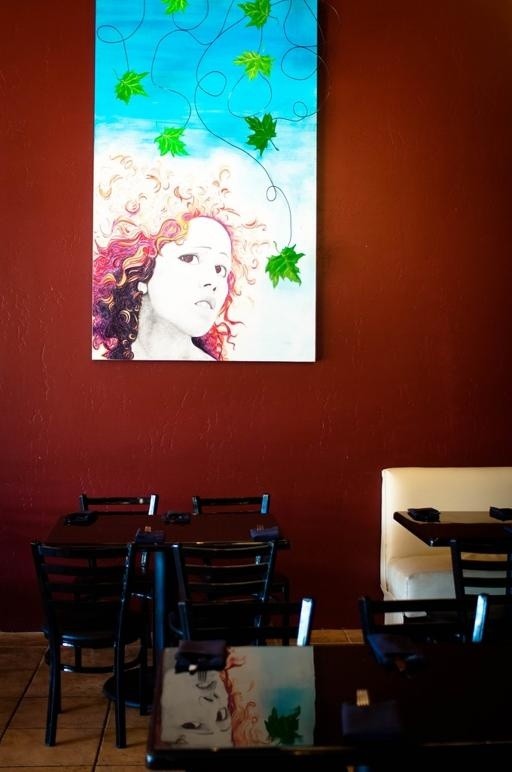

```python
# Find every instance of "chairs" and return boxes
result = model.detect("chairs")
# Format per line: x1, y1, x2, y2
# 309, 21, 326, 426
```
357, 465, 511, 642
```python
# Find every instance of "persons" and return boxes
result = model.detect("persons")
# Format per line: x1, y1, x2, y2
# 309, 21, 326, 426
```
93, 205, 244, 363
163, 645, 270, 749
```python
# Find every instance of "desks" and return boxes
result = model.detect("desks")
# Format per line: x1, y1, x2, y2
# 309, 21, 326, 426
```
144, 642, 512, 771
